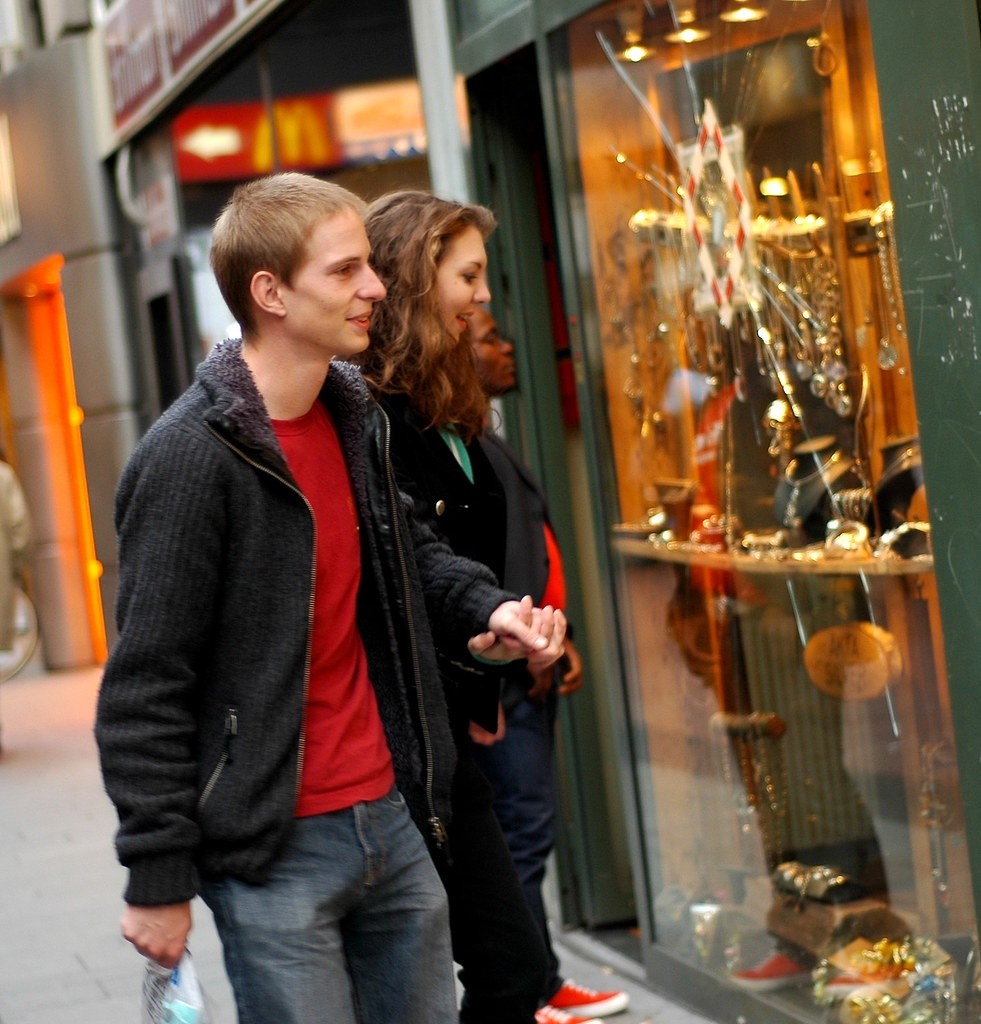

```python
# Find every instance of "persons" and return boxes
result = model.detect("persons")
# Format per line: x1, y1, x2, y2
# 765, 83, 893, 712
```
0, 447, 31, 761
461, 303, 631, 1024
94, 174, 565, 1024
365, 191, 547, 1024
669, 367, 905, 997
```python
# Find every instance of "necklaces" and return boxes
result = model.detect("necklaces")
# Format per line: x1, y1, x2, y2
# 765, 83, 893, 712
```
626, 202, 907, 417
879, 446, 920, 481
783, 450, 841, 526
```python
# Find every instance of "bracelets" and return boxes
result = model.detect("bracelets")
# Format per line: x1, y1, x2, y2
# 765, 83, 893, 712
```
833, 489, 873, 524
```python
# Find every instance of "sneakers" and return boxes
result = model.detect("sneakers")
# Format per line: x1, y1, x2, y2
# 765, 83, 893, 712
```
729, 954, 816, 992
535, 1005, 602, 1024
814, 961, 919, 1007
545, 978, 629, 1017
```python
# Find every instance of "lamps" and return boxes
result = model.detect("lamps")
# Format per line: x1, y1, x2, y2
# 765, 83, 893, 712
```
618, 0, 773, 62
